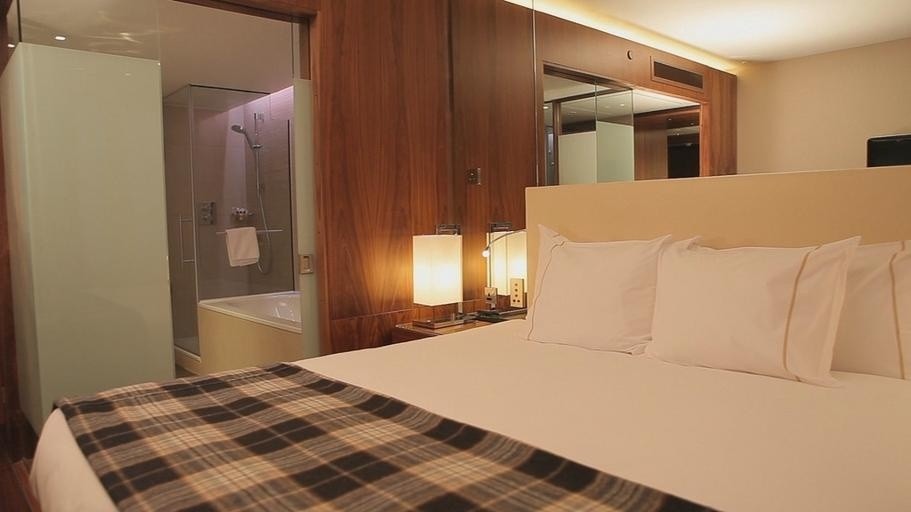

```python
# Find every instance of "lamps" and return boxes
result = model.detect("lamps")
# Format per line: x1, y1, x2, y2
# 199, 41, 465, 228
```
411, 221, 467, 329
479, 218, 526, 322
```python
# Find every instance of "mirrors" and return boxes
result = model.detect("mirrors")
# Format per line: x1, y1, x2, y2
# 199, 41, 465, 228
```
446, 0, 911, 320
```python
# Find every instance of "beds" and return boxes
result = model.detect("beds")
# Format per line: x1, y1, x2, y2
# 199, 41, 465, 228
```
26, 161, 911, 511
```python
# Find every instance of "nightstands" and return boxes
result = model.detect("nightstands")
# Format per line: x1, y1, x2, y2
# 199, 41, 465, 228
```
388, 314, 491, 344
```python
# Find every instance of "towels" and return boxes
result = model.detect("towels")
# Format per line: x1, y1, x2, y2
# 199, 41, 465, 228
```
225, 225, 259, 268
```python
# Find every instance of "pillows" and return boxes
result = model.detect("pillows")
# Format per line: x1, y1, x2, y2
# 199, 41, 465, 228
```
641, 233, 863, 384
520, 220, 672, 358
828, 235, 910, 383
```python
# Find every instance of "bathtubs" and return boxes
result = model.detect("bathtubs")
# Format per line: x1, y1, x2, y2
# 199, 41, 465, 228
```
198, 290, 303, 376
